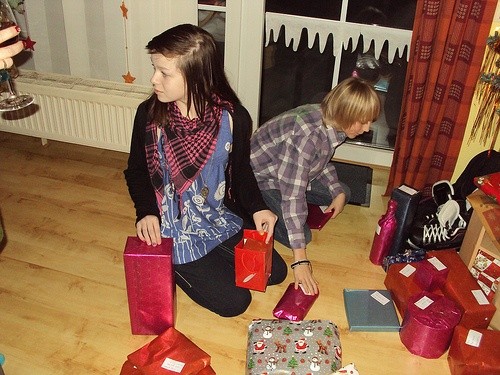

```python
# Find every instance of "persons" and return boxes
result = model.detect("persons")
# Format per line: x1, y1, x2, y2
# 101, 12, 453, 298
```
0, 24, 33, 375
250, 76, 381, 295
406, 150, 500, 252
123, 24, 288, 317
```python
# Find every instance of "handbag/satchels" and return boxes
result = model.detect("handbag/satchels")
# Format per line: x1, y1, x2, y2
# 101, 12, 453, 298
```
235, 229, 273, 293
403, 180, 471, 251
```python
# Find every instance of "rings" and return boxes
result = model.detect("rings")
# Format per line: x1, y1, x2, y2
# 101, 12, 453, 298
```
1, 59, 7, 70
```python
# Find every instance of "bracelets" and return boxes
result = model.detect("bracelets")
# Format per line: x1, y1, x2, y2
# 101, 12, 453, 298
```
290, 259, 312, 269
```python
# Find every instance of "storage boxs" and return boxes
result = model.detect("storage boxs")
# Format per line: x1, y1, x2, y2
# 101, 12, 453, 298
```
459, 209, 500, 330
123, 236, 177, 335
343, 248, 500, 375
389, 183, 420, 256
246, 319, 342, 375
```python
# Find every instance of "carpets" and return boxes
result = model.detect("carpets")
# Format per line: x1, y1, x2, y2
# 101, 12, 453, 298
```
330, 160, 373, 206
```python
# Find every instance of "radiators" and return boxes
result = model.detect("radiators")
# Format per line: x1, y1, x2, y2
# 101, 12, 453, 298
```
0, 82, 145, 154
